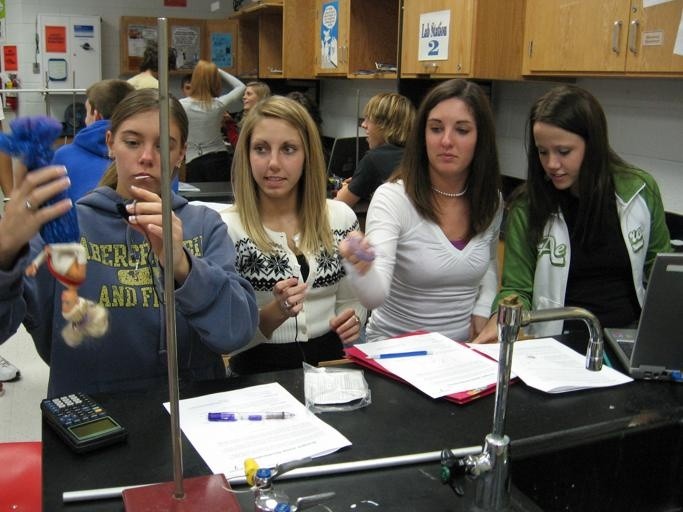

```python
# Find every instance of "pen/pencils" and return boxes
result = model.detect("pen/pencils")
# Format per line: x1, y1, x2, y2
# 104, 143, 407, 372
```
209, 412, 297, 421
603, 350, 612, 368
366, 350, 432, 359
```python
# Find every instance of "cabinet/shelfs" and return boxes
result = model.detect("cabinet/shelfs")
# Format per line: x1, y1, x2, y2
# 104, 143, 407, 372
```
35, 12, 103, 96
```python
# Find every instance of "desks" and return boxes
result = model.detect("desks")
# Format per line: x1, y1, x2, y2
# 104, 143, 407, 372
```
42, 331, 682, 511
177, 171, 372, 232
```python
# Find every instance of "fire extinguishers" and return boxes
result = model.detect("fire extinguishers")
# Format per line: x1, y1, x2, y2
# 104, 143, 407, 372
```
5, 73, 20, 112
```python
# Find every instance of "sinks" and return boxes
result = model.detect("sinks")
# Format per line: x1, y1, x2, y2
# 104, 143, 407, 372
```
506, 414, 682, 512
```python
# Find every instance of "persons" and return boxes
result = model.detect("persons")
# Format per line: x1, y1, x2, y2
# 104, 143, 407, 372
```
469, 84, 671, 344
41, 43, 419, 245
0, 94, 22, 397
338, 78, 505, 344
216, 94, 368, 379
0, 87, 260, 399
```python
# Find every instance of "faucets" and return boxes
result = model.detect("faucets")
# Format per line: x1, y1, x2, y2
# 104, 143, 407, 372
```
478, 290, 605, 512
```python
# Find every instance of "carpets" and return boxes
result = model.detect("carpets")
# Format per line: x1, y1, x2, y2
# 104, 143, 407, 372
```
0, 442, 42, 512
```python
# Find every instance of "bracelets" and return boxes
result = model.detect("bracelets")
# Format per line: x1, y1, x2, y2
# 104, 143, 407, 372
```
3, 197, 11, 201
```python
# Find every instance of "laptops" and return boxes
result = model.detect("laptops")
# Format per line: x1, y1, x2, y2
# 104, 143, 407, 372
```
602, 251, 682, 382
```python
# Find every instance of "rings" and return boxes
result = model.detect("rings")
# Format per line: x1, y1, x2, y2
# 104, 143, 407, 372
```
283, 300, 292, 308
24, 199, 32, 208
356, 318, 359, 324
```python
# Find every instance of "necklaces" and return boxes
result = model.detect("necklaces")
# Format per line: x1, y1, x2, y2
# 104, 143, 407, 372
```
430, 182, 469, 197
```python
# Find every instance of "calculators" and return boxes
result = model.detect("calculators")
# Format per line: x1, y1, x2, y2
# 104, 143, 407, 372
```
40, 392, 129, 455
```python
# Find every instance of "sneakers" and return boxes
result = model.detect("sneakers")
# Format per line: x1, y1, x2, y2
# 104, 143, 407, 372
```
0, 355, 21, 382
